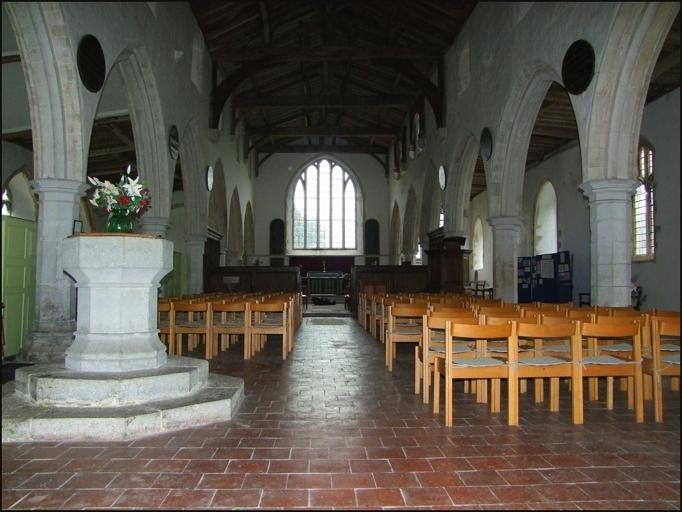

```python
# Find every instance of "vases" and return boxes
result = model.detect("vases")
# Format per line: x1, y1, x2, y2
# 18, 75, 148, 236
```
104, 207, 134, 232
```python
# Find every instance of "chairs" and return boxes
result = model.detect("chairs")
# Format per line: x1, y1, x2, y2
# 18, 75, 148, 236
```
158, 290, 301, 363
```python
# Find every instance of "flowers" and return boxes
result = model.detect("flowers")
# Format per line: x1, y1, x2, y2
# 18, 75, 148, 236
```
88, 164, 153, 218
